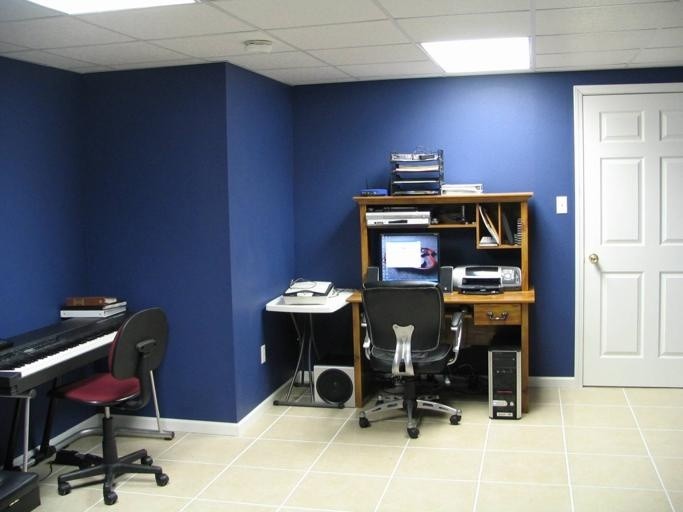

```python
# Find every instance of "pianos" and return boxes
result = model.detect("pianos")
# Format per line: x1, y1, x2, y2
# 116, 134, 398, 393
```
1, 313, 132, 395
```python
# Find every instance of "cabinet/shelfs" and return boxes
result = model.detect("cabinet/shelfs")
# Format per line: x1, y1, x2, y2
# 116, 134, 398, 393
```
352, 192, 534, 290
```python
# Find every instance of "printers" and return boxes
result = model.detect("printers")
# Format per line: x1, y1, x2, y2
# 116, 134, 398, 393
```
453, 265, 522, 296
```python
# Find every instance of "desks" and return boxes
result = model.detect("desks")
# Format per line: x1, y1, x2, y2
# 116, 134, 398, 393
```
345, 286, 535, 413
265, 287, 355, 409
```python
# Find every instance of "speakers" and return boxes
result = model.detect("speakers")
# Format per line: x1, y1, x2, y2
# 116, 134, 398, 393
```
366, 266, 379, 285
440, 265, 453, 292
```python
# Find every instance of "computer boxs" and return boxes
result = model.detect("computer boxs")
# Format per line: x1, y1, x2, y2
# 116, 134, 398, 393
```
487, 337, 522, 420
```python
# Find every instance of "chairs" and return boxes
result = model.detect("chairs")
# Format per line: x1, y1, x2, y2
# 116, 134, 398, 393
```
359, 280, 463, 436
45, 306, 170, 505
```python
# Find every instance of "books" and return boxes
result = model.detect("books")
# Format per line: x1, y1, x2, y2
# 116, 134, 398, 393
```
479, 201, 513, 245
57, 305, 127, 319
53, 293, 126, 311
359, 146, 486, 196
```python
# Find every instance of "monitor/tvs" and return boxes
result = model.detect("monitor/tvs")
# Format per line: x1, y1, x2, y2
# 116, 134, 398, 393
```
376, 227, 442, 288
